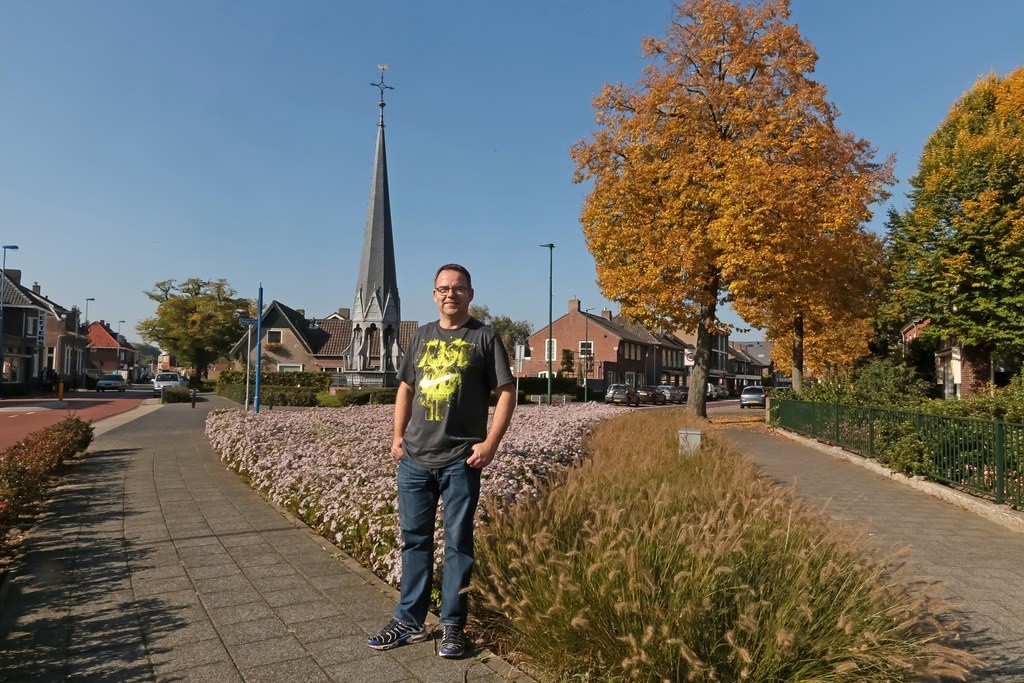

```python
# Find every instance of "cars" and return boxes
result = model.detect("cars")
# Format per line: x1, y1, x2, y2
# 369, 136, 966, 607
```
604, 384, 641, 408
150, 371, 182, 397
678, 385, 689, 401
96, 374, 126, 393
739, 386, 790, 410
706, 383, 718, 402
656, 385, 684, 404
636, 385, 667, 405
715, 384, 729, 399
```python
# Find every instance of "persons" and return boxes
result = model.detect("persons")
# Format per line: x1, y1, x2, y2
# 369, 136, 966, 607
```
40, 366, 58, 392
365, 265, 516, 659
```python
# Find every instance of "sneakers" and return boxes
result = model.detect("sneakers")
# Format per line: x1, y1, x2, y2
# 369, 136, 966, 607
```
438, 624, 466, 658
366, 618, 428, 651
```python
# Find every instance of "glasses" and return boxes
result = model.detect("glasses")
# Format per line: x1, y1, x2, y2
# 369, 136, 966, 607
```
436, 287, 469, 296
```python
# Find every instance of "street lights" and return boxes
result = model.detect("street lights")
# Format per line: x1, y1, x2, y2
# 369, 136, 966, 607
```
81, 297, 96, 389
585, 306, 596, 402
0, 244, 19, 322
117, 320, 126, 375
539, 242, 556, 405
